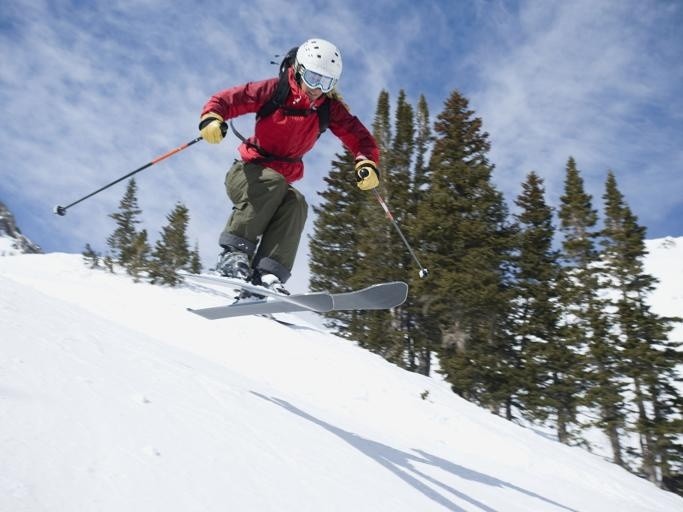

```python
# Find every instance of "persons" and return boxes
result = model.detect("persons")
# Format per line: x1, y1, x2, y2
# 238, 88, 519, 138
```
197, 38, 379, 304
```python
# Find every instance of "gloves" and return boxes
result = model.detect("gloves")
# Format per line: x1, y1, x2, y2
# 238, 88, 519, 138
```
354, 160, 380, 190
199, 112, 228, 143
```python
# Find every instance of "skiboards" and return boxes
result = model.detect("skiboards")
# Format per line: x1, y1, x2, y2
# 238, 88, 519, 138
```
175, 267, 409, 319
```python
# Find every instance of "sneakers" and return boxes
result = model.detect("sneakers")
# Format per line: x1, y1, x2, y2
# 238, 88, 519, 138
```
216, 251, 250, 280
240, 269, 290, 298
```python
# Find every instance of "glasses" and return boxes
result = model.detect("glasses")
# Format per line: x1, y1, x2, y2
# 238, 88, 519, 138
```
294, 57, 339, 93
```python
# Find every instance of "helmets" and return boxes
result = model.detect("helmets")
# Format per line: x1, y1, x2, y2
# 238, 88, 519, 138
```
293, 39, 342, 94
